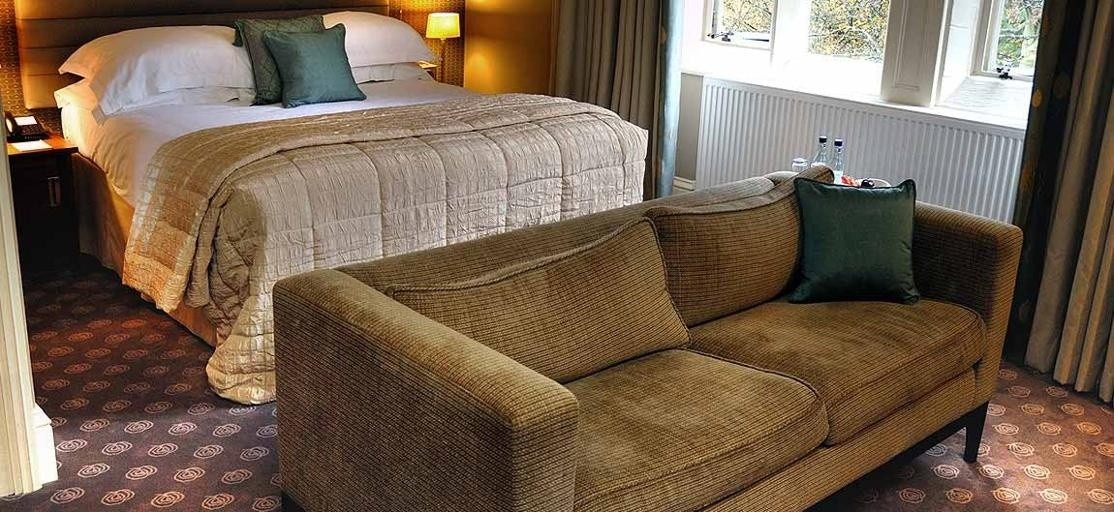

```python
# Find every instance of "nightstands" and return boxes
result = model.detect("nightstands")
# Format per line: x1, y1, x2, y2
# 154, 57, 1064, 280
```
7, 134, 81, 252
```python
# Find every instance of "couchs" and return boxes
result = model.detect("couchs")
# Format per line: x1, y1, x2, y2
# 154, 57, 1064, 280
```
271, 171, 1026, 510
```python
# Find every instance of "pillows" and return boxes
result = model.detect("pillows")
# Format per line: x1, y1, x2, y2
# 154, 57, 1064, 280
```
54, 77, 257, 123
234, 15, 328, 106
261, 21, 367, 109
350, 61, 440, 86
784, 177, 921, 307
322, 10, 441, 69
384, 217, 695, 381
55, 25, 254, 116
639, 165, 834, 327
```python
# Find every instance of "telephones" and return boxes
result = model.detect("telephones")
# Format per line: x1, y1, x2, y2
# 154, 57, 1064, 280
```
4, 110, 45, 139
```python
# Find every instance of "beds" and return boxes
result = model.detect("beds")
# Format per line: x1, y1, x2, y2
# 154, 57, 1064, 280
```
14, 1, 649, 348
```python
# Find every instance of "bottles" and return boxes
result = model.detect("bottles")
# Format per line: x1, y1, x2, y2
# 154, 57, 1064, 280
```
792, 136, 843, 188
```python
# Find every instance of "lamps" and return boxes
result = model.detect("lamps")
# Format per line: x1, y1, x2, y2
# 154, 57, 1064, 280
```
425, 14, 462, 43
